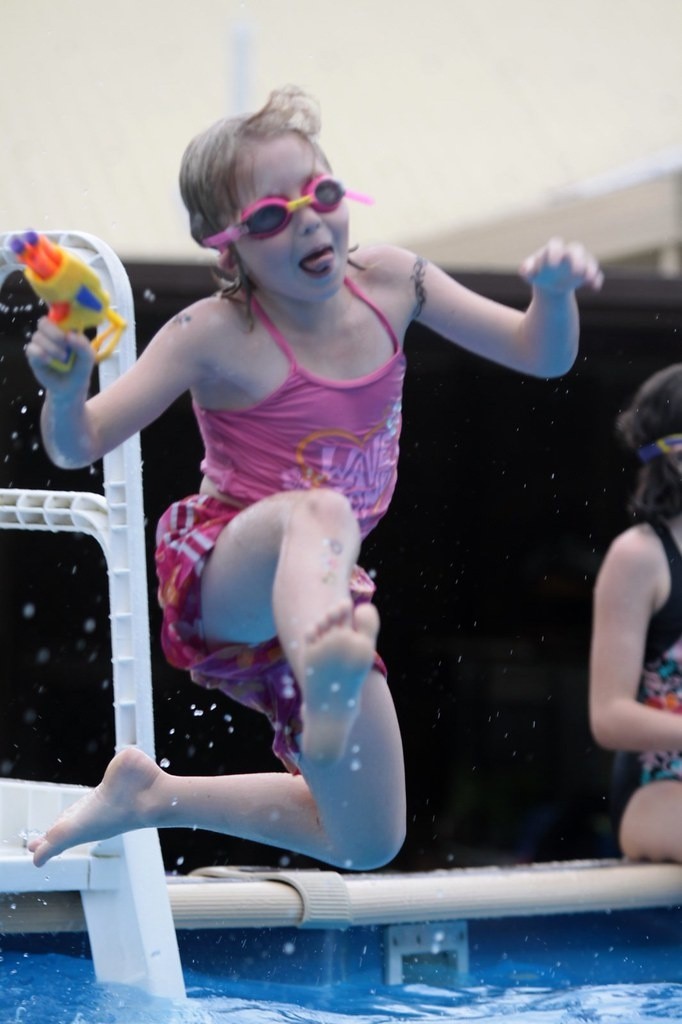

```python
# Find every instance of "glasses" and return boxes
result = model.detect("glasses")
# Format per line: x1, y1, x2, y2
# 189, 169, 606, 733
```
204, 176, 344, 246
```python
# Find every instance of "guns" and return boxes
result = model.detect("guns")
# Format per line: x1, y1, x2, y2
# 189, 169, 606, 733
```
7, 227, 126, 377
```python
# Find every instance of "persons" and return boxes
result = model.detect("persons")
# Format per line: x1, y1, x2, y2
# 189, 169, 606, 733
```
587, 367, 682, 858
24, 79, 605, 868
